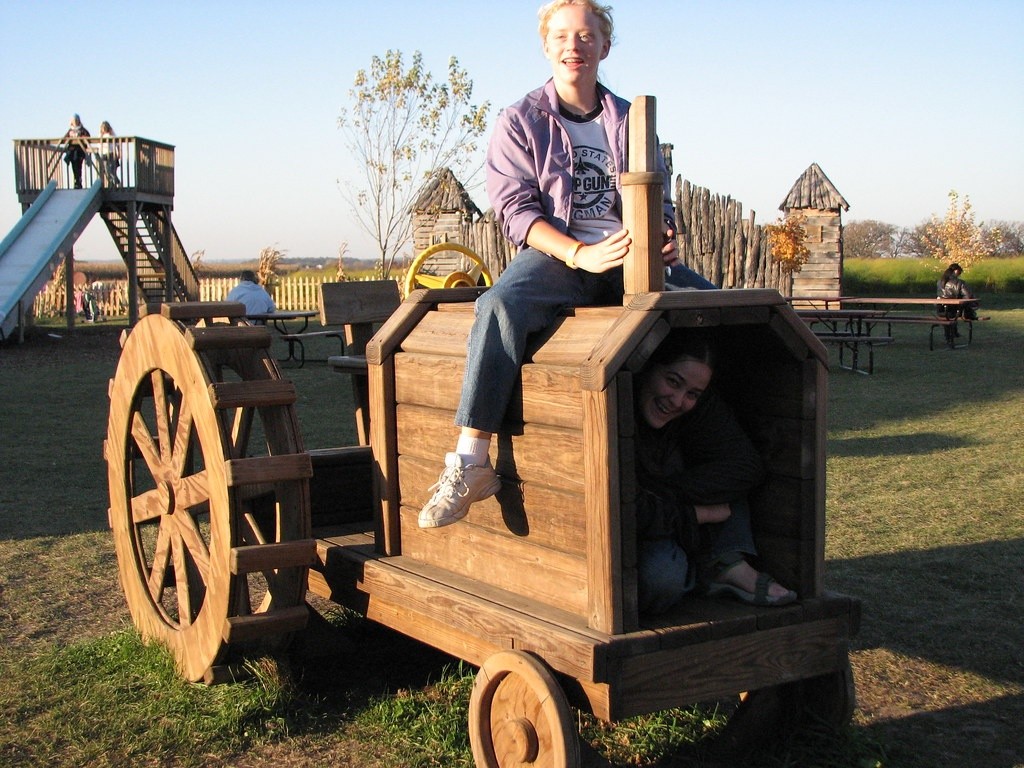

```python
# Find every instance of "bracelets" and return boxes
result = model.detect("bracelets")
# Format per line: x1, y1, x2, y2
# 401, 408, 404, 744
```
566, 240, 586, 270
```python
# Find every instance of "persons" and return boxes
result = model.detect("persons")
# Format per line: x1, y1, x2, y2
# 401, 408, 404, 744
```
225, 270, 275, 326
634, 332, 797, 609
62, 113, 91, 190
417, 1, 721, 529
98, 121, 120, 189
936, 263, 979, 345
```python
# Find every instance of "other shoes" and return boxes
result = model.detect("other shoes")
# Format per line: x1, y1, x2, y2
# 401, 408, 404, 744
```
954, 328, 961, 337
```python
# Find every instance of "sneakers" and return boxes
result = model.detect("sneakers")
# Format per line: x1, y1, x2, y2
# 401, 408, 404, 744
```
418, 452, 502, 528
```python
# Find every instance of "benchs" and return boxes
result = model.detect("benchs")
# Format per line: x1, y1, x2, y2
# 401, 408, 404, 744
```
788, 304, 991, 377
279, 330, 344, 369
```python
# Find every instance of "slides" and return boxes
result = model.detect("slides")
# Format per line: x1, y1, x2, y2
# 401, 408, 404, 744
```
0, 174, 104, 344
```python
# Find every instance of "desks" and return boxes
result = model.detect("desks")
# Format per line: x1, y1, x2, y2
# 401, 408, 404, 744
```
246, 310, 320, 362
794, 310, 888, 375
841, 298, 980, 351
784, 297, 852, 337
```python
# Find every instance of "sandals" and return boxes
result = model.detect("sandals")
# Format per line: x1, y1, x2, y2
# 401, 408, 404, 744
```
704, 554, 797, 608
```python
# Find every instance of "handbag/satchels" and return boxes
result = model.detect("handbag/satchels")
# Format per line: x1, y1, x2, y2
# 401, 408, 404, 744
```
958, 282, 979, 318
